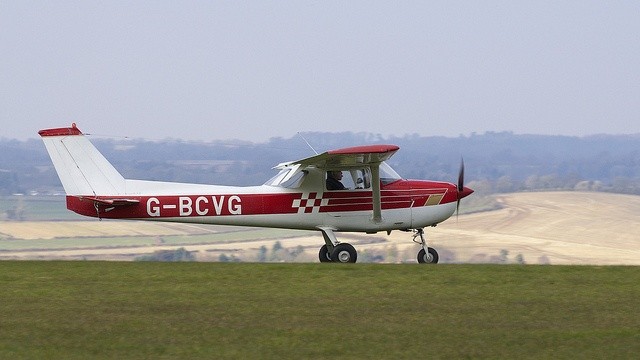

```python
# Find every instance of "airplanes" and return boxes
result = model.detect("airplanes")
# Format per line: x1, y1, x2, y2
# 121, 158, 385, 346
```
38, 123, 474, 264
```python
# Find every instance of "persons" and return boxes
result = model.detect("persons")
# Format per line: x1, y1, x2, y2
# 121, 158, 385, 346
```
325, 171, 350, 191
